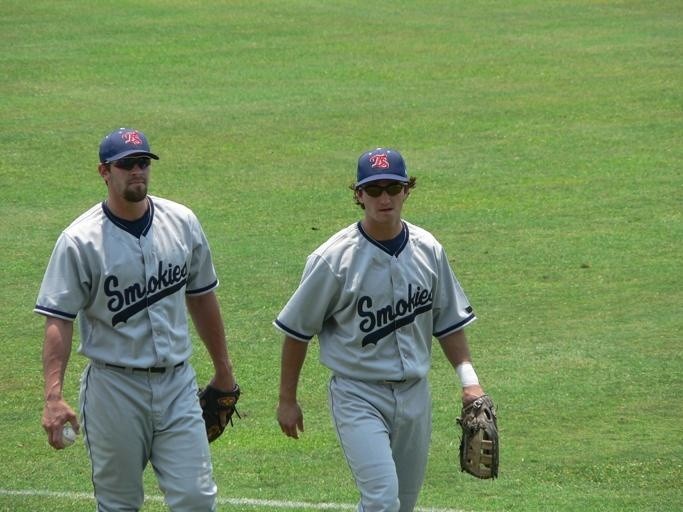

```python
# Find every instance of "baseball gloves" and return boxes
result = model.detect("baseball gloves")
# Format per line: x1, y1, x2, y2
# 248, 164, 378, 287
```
455, 395, 499, 479
197, 383, 240, 443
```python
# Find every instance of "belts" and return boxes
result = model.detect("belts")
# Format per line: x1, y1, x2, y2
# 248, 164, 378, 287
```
105, 362, 184, 373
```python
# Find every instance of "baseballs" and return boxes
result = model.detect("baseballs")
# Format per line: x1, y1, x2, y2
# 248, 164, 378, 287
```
61, 425, 75, 447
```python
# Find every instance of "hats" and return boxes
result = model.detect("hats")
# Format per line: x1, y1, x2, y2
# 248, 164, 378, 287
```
356, 148, 408, 187
99, 128, 159, 164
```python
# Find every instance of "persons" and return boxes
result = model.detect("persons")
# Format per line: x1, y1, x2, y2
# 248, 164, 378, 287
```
30, 126, 244, 511
269, 143, 501, 511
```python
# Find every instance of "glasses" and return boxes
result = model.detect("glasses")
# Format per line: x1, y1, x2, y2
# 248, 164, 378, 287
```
359, 181, 404, 197
104, 156, 151, 170
